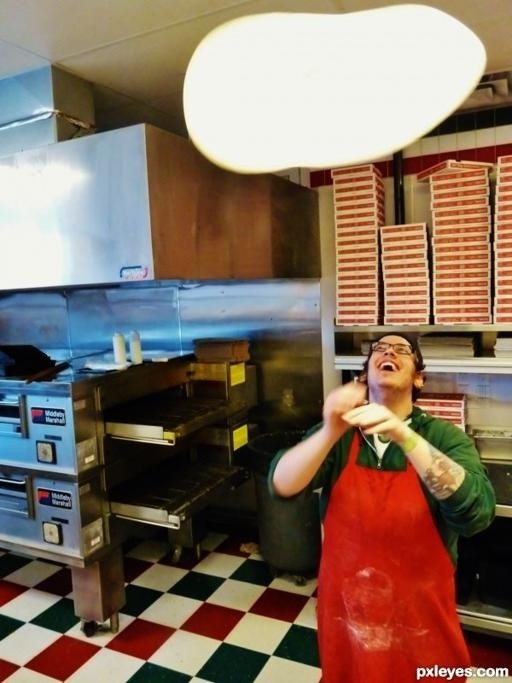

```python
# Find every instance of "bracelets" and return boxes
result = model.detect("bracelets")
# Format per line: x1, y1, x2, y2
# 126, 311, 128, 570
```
393, 431, 421, 456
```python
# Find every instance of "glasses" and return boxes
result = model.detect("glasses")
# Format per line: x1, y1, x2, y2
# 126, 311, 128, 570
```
372, 342, 419, 364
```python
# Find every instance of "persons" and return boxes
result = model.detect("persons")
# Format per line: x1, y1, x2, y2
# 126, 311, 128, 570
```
266, 329, 501, 682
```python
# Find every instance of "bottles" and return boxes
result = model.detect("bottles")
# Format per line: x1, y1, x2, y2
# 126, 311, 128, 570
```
129, 330, 143, 364
112, 331, 127, 364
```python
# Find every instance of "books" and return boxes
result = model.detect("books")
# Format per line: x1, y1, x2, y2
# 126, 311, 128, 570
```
357, 332, 511, 364
191, 336, 251, 365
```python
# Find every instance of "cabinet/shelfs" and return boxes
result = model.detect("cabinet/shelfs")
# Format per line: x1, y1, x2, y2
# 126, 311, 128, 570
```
321, 322, 512, 640
